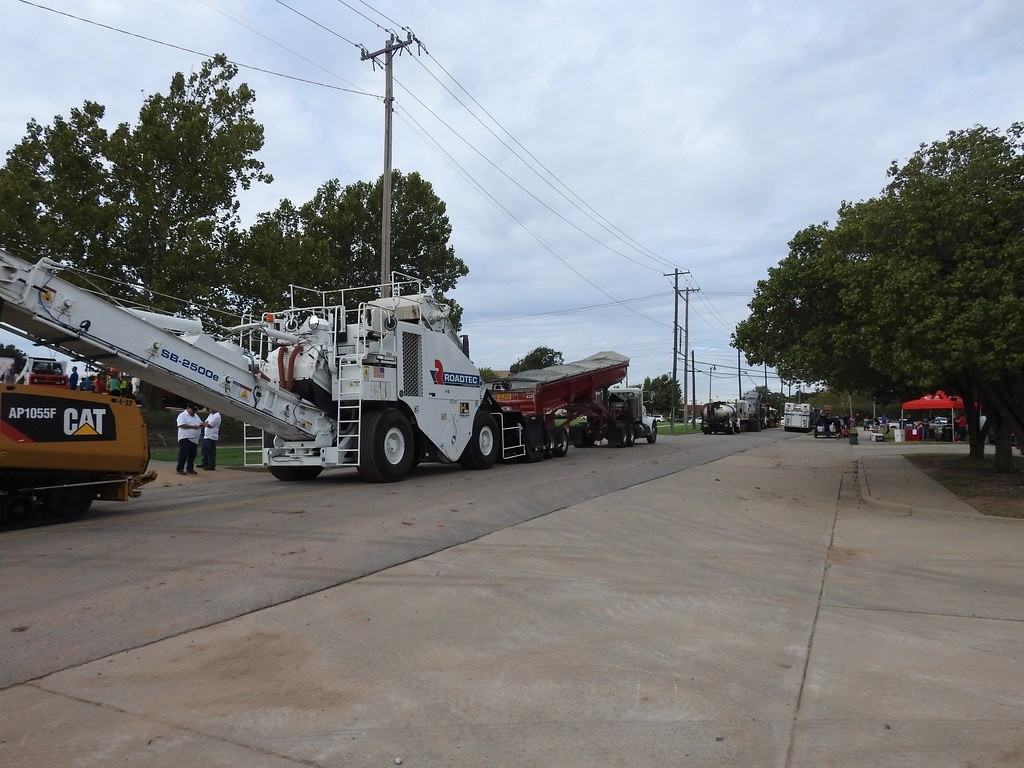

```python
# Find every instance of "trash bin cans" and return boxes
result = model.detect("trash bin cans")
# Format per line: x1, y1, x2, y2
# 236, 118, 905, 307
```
894, 428, 906, 442
848, 432, 859, 445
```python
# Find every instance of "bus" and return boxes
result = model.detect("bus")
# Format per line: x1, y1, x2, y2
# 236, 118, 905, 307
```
0, 250, 526, 483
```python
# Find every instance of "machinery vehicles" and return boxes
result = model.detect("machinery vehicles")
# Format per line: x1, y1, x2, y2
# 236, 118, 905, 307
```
0, 357, 158, 530
484, 351, 658, 461
701, 392, 778, 435
784, 402, 822, 433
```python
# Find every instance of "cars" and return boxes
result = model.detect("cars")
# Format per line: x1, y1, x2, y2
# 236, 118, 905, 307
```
648, 409, 702, 424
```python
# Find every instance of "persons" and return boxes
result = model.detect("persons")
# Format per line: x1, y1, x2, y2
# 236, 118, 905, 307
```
832, 413, 968, 443
176, 405, 221, 475
68, 366, 133, 398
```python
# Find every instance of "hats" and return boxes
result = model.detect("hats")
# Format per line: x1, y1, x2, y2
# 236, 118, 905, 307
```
188, 403, 198, 410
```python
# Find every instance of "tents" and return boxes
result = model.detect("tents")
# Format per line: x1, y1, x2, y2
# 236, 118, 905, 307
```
900, 390, 964, 444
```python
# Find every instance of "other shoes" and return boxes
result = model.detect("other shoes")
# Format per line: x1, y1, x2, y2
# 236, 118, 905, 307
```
187, 468, 197, 474
177, 469, 186, 475
203, 467, 215, 470
197, 464, 205, 468
